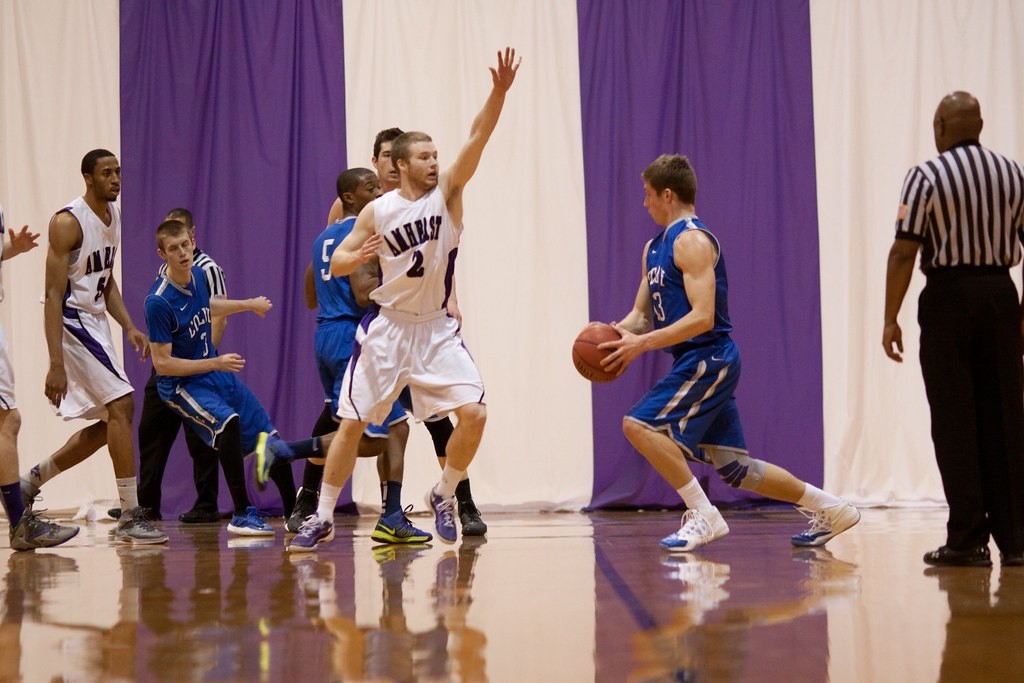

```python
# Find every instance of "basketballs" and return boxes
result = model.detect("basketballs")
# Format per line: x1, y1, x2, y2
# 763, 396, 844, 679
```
572, 324, 630, 383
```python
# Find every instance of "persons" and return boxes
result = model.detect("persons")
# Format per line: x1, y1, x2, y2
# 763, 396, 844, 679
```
19, 150, 172, 545
283, 48, 525, 556
255, 126, 494, 540
619, 548, 860, 683
0, 534, 491, 682
925, 563, 1023, 683
104, 206, 226, 523
143, 218, 298, 533
0, 207, 82, 549
597, 146, 861, 551
879, 88, 1023, 570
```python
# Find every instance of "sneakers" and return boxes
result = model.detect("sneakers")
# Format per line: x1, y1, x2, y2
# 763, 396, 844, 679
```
794, 498, 862, 547
0, 479, 43, 517
288, 552, 336, 583
179, 505, 219, 523
293, 514, 336, 552
458, 500, 488, 535
372, 538, 433, 564
999, 548, 1024, 565
227, 503, 276, 536
426, 486, 458, 544
285, 485, 321, 533
371, 505, 433, 544
10, 507, 84, 551
109, 502, 162, 522
658, 505, 730, 550
114, 507, 169, 546
284, 533, 300, 547
923, 537, 991, 566
255, 430, 279, 489
